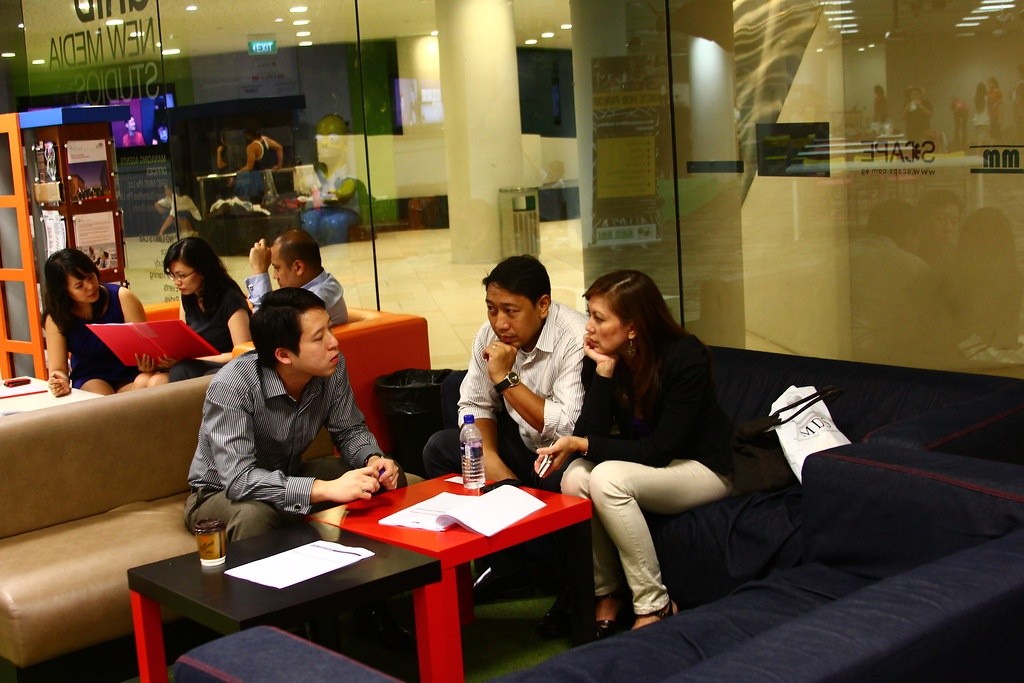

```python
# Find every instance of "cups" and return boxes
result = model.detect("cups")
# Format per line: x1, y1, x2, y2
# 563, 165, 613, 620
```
194, 518, 226, 567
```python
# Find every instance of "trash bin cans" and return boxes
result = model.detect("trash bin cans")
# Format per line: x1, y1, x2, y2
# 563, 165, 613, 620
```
375, 368, 453, 480
498, 187, 540, 260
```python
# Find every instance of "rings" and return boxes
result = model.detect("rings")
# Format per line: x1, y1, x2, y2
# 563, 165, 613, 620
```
493, 344, 497, 349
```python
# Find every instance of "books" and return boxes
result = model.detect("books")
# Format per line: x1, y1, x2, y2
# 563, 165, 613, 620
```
378, 485, 546, 538
86, 319, 221, 366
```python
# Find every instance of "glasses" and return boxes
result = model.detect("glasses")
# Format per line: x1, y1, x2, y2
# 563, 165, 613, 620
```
169, 270, 196, 280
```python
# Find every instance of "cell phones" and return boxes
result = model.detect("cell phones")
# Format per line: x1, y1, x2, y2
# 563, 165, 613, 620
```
537, 441, 555, 477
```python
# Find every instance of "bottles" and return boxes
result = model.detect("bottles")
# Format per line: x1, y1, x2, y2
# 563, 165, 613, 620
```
459, 415, 486, 489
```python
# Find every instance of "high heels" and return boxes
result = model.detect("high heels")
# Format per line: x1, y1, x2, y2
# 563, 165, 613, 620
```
593, 591, 628, 641
631, 600, 675, 631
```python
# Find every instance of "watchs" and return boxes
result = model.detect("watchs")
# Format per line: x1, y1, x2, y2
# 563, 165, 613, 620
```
494, 371, 520, 394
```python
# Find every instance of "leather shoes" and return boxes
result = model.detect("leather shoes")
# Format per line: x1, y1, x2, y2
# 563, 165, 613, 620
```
473, 568, 528, 600
535, 587, 583, 640
352, 603, 416, 652
308, 619, 340, 653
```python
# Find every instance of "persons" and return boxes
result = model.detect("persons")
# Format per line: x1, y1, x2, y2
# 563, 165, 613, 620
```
534, 269, 732, 642
89, 247, 110, 269
154, 182, 201, 239
852, 64, 1024, 353
421, 255, 587, 637
185, 286, 415, 650
77, 184, 103, 200
227, 124, 283, 203
132, 237, 253, 389
245, 230, 348, 327
217, 129, 244, 171
41, 248, 147, 397
158, 127, 167, 141
123, 114, 145, 146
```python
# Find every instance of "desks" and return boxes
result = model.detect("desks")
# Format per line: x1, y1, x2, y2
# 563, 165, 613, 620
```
304, 471, 595, 682
125, 519, 452, 681
0, 376, 106, 420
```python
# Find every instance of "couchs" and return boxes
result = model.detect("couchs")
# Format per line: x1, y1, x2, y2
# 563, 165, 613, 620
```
0, 301, 1024, 682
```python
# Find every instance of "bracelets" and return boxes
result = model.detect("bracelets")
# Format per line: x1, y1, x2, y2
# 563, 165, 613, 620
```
363, 452, 385, 467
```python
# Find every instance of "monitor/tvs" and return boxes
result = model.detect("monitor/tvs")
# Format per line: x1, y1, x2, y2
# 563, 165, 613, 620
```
109, 92, 175, 158
